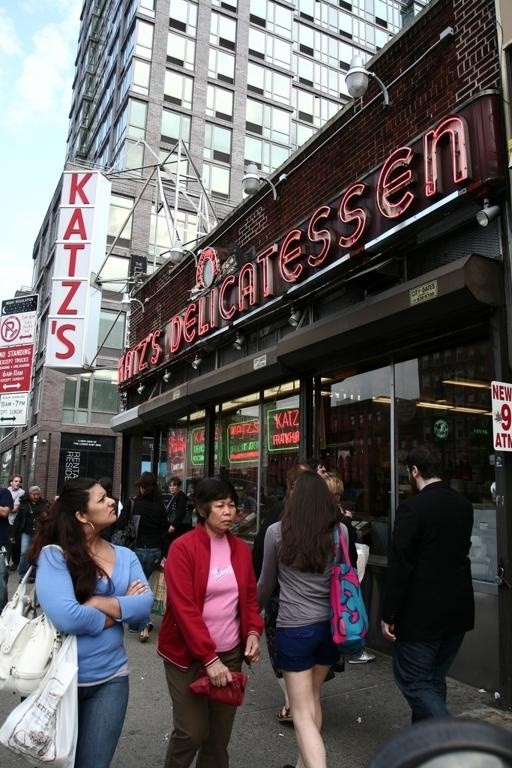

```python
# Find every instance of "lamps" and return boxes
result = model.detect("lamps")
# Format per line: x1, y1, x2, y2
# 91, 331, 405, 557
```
121, 293, 144, 315
242, 161, 279, 205
233, 333, 245, 351
163, 369, 171, 383
192, 353, 202, 369
476, 197, 500, 226
288, 306, 302, 327
345, 56, 389, 109
137, 381, 145, 394
170, 238, 198, 270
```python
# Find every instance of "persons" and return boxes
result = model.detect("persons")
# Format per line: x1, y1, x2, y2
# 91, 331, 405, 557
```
322, 477, 377, 664
129, 472, 168, 642
19, 486, 49, 583
6, 475, 25, 569
157, 478, 264, 767
251, 464, 314, 723
97, 477, 123, 542
255, 470, 351, 768
381, 444, 475, 724
166, 477, 187, 537
30, 476, 155, 767
0, 488, 14, 614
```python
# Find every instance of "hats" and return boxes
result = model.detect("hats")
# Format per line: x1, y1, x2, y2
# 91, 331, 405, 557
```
0, 488, 14, 510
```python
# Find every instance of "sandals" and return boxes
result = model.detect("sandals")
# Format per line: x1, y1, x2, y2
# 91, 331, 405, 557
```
275, 707, 293, 723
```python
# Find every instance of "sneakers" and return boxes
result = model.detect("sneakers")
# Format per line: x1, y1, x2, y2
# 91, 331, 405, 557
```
348, 651, 376, 664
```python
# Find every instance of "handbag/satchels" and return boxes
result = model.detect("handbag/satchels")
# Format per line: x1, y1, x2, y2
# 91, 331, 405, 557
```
332, 561, 369, 645
123, 522, 135, 546
0, 594, 64, 699
146, 569, 167, 616
